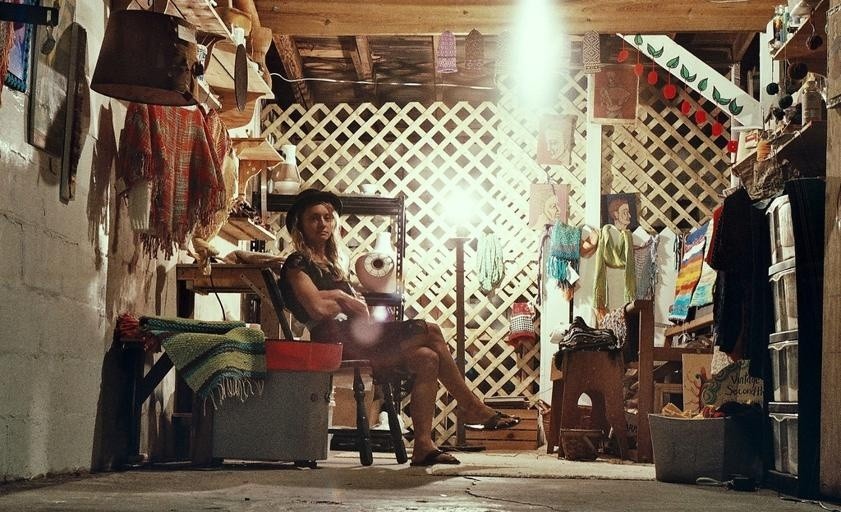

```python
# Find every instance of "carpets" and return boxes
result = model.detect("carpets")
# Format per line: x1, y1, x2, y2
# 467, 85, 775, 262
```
431, 450, 656, 480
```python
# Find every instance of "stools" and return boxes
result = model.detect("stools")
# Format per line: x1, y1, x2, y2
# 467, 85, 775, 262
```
547, 349, 630, 460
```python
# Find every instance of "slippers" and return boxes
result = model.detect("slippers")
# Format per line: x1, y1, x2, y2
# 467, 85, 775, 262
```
410, 449, 458, 466
463, 411, 520, 430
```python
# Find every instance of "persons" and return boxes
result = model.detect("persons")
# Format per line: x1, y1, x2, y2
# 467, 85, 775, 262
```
607, 196, 632, 230
281, 187, 521, 467
532, 185, 561, 228
599, 66, 633, 118
544, 118, 568, 162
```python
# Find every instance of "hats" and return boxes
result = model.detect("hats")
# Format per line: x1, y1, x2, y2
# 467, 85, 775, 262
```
286, 188, 343, 235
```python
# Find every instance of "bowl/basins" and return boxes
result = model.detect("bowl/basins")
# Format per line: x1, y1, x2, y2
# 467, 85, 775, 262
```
265, 338, 343, 372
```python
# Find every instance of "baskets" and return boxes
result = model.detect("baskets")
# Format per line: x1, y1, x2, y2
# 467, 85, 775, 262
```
648, 414, 731, 485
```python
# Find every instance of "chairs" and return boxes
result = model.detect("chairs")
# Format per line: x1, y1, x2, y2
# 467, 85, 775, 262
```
262, 268, 408, 465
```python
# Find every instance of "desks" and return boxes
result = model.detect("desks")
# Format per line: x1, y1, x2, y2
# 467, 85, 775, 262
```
626, 299, 714, 463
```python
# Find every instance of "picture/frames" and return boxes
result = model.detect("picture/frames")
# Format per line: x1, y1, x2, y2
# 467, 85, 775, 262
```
0, 1, 35, 92
